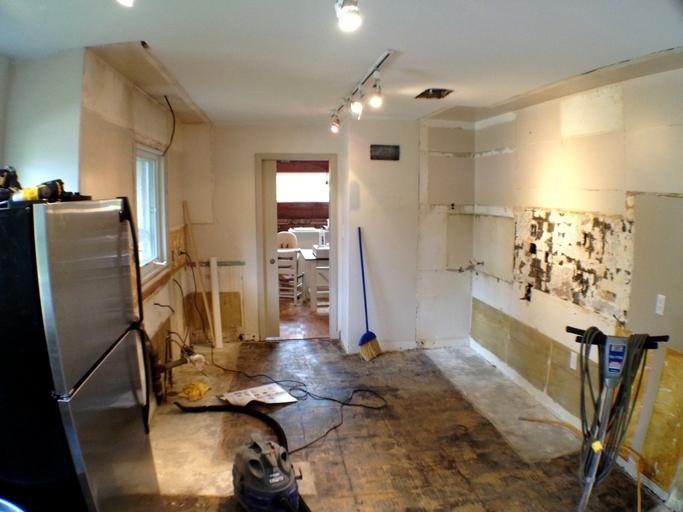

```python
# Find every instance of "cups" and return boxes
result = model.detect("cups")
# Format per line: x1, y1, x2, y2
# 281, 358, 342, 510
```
319, 228, 326, 247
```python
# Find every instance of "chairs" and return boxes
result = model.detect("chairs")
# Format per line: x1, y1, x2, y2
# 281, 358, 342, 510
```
277, 231, 297, 248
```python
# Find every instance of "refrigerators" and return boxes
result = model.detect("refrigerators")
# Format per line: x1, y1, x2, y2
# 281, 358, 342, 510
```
0, 197, 170, 511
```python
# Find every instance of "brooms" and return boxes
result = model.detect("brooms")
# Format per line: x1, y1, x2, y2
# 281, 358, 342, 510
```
357, 225, 383, 361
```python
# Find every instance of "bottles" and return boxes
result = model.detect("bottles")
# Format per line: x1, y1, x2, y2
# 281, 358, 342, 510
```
281, 241, 289, 248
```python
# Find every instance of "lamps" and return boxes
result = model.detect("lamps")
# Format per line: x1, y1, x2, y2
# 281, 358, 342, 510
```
334, 0, 362, 33
328, 49, 393, 136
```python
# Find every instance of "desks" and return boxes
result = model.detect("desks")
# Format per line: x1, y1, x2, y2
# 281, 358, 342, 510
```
287, 227, 319, 248
300, 248, 330, 313
